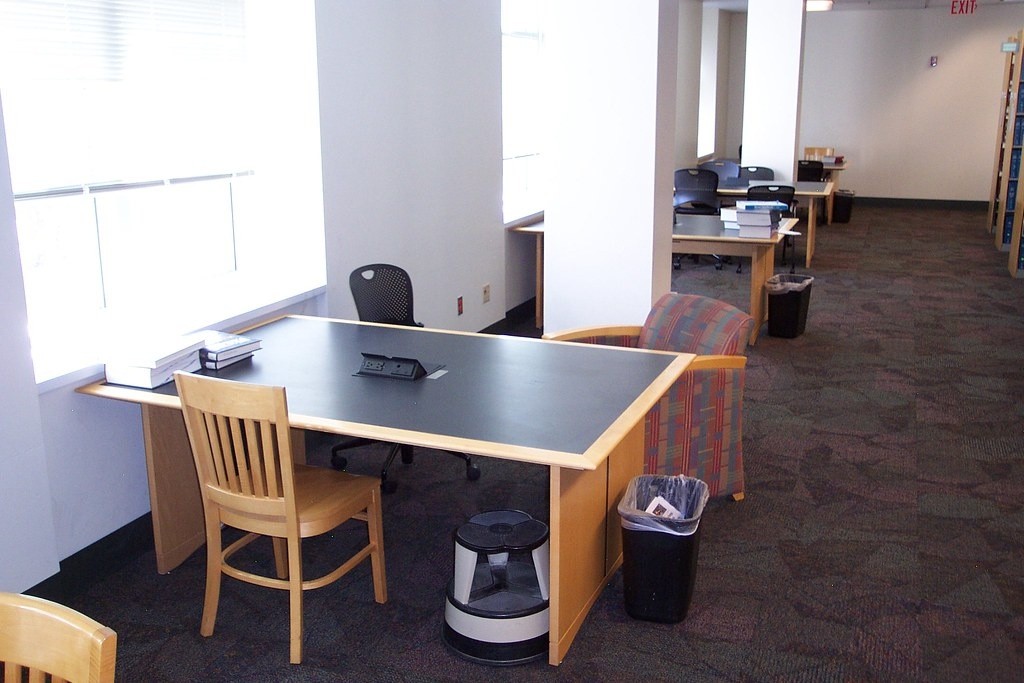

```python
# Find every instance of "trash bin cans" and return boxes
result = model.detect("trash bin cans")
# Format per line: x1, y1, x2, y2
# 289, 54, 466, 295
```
615, 474, 709, 624
767, 273, 814, 339
833, 189, 855, 223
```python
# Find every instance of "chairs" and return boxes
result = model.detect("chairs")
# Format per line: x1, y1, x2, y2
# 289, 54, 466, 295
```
0, 592, 117, 683
173, 370, 389, 664
671, 145, 835, 274
330, 263, 481, 493
541, 292, 755, 502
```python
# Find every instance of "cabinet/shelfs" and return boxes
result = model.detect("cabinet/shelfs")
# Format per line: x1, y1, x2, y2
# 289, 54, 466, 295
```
987, 30, 1024, 278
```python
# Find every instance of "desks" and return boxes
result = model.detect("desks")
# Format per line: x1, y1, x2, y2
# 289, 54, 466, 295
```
698, 157, 847, 225
672, 180, 834, 269
506, 212, 799, 346
74, 314, 698, 667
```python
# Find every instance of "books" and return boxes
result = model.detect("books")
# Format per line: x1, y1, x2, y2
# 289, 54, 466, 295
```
105, 326, 263, 391
720, 196, 788, 239
822, 154, 844, 167
993, 51, 1024, 270
